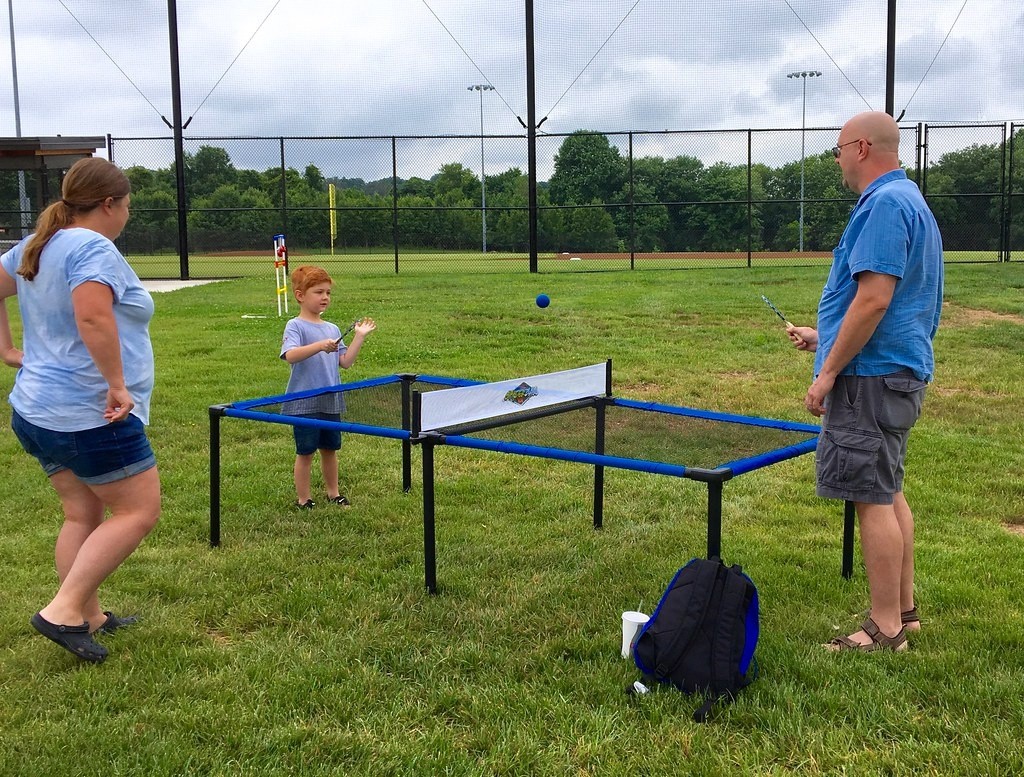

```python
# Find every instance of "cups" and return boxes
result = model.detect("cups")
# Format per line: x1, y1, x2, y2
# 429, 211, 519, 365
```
621, 612, 650, 659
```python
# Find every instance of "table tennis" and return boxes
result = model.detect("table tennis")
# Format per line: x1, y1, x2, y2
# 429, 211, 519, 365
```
535, 293, 550, 308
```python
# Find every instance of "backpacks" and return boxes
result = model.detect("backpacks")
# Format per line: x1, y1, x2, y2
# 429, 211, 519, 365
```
626, 555, 760, 723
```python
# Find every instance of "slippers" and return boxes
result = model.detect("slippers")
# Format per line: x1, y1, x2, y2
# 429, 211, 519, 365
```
30, 612, 108, 664
89, 611, 137, 636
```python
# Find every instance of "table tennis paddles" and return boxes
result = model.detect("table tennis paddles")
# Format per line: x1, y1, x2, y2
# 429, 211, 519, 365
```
327, 318, 361, 354
760, 295, 805, 343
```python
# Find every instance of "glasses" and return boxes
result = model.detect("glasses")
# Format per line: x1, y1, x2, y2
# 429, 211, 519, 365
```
832, 139, 872, 158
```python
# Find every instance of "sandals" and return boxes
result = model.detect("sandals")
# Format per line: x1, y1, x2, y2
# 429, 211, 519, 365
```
869, 606, 919, 623
821, 618, 906, 654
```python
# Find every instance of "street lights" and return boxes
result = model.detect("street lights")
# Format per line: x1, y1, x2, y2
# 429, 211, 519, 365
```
467, 83, 495, 254
787, 70, 823, 253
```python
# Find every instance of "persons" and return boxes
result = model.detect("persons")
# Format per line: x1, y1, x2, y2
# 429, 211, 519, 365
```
786, 112, 945, 652
279, 265, 377, 509
0, 157, 161, 662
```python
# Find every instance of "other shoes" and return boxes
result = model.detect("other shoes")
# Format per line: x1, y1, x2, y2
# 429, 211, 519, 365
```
327, 495, 349, 505
298, 499, 316, 510
